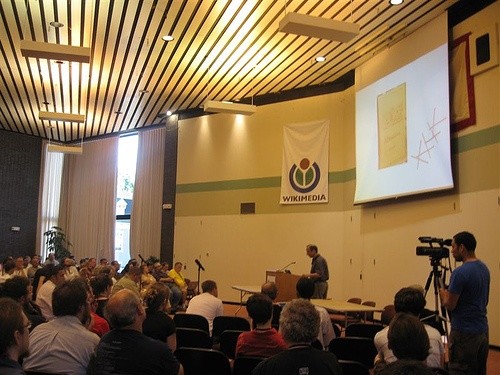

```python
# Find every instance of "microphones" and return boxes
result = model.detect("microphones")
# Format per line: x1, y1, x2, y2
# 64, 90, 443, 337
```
195, 259, 205, 271
138, 254, 144, 262
279, 262, 296, 272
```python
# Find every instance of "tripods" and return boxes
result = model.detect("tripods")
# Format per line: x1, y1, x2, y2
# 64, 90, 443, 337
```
416, 266, 452, 341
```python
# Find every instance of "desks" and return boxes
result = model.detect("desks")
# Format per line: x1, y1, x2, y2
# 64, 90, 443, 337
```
231, 285, 261, 316
310, 298, 385, 328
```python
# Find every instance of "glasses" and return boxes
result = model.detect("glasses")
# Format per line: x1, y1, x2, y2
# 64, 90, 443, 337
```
24, 322, 31, 328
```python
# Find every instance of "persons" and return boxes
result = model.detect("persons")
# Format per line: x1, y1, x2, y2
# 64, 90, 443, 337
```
375, 313, 446, 375
374, 287, 445, 369
0, 253, 185, 336
186, 280, 224, 345
252, 299, 343, 375
0, 297, 32, 375
236, 295, 289, 356
88, 289, 184, 375
305, 244, 329, 299
296, 278, 336, 350
262, 281, 277, 303
440, 232, 490, 375
23, 282, 101, 375
142, 282, 177, 353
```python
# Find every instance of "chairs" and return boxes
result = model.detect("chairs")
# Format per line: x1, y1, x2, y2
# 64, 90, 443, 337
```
172, 298, 446, 375
